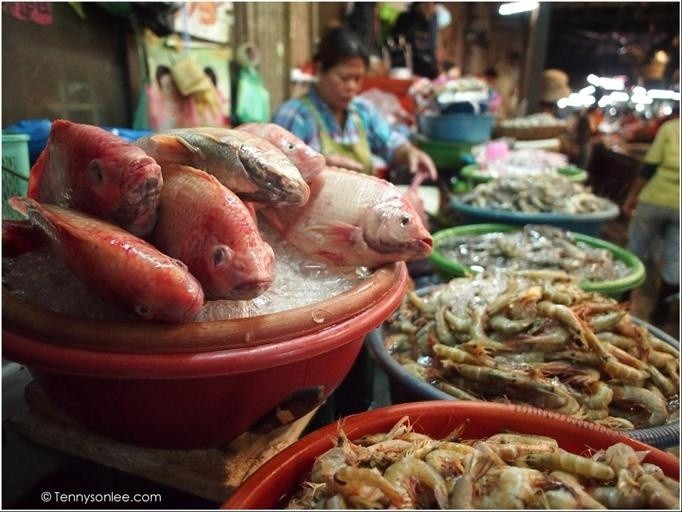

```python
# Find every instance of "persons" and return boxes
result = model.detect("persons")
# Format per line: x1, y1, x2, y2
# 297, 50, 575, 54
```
185, 67, 230, 127
271, 26, 438, 189
153, 65, 186, 128
382, 2, 440, 81
619, 117, 681, 328
478, 65, 501, 115
432, 60, 460, 87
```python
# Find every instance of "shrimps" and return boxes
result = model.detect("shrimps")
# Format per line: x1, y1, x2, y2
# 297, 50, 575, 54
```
287, 413, 680, 509
384, 271, 679, 430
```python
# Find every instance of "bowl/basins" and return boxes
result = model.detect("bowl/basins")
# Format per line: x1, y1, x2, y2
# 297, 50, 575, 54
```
414, 115, 494, 143
364, 282, 679, 448
423, 135, 645, 303
220, 398, 679, 509
2, 262, 406, 449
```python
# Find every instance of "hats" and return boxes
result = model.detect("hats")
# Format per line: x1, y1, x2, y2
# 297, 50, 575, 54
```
540, 69, 573, 103
